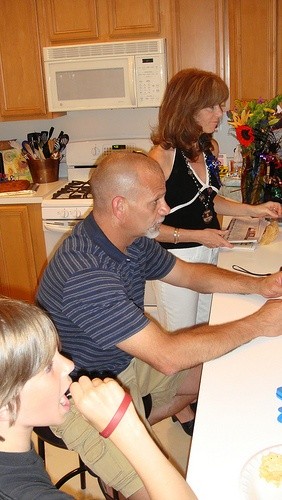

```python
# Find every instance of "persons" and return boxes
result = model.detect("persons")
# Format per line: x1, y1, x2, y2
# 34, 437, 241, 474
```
145, 68, 282, 435
1, 294, 197, 500
32, 150, 282, 500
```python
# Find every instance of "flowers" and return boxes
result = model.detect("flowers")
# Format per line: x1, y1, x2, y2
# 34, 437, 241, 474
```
226, 95, 282, 206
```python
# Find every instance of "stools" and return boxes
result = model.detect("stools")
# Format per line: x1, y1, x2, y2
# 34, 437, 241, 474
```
33, 393, 152, 490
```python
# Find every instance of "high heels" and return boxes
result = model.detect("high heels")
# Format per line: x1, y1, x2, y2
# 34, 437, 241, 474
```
173, 413, 195, 436
189, 400, 197, 412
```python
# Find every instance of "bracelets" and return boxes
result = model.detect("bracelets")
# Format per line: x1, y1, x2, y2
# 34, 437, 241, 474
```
174, 226, 180, 246
98, 392, 132, 439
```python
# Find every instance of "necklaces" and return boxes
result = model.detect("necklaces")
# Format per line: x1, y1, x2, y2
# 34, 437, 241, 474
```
181, 146, 212, 225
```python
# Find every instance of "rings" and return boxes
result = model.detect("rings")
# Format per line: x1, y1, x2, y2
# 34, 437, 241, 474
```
273, 207, 276, 211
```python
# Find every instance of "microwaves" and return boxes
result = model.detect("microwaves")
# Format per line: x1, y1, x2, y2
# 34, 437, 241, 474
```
42, 38, 168, 113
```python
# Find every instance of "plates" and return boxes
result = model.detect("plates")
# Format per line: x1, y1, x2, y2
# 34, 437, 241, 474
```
238, 445, 282, 500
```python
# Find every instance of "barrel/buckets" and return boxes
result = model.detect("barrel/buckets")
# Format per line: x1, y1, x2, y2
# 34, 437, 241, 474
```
25, 158, 60, 184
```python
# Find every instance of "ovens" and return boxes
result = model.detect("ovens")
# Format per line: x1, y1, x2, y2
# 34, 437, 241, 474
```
42, 220, 81, 264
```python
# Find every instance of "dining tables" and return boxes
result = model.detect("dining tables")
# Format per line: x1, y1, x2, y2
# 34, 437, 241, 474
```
185, 214, 282, 500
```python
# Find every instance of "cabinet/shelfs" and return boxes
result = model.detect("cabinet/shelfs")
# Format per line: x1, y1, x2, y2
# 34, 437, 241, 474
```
0, 204, 47, 305
0, 0, 282, 121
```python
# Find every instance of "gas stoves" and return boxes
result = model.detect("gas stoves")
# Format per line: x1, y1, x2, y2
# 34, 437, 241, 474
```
40, 137, 155, 219
